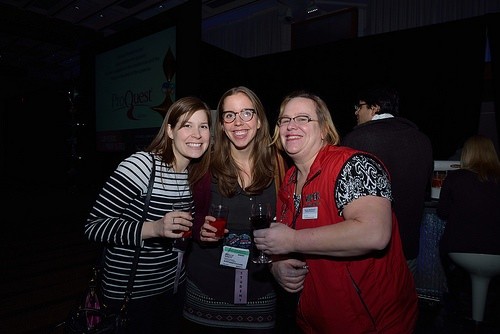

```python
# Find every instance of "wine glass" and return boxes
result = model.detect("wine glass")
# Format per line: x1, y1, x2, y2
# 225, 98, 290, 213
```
248, 202, 274, 264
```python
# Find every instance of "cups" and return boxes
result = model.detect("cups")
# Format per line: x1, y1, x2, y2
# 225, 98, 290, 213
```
209, 204, 228, 239
432, 172, 446, 187
172, 201, 193, 239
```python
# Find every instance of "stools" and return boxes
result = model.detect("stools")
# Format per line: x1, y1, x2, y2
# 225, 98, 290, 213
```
448, 252, 500, 334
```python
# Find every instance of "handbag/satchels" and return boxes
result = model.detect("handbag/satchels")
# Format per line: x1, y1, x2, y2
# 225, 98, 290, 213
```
64, 272, 132, 334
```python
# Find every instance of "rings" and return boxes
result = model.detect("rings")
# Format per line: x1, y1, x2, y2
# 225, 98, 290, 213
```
173, 217, 175, 223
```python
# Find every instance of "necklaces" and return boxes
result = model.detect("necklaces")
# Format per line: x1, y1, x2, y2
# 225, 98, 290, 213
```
171, 164, 188, 204
294, 180, 297, 198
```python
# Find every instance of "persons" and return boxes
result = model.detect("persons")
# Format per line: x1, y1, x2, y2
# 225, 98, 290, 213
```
177, 87, 289, 334
82, 96, 213, 333
339, 83, 432, 280
437, 134, 500, 281
253, 89, 420, 334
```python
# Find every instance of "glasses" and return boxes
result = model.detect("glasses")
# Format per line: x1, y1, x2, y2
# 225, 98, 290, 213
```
276, 115, 322, 126
222, 107, 257, 123
353, 101, 376, 109
